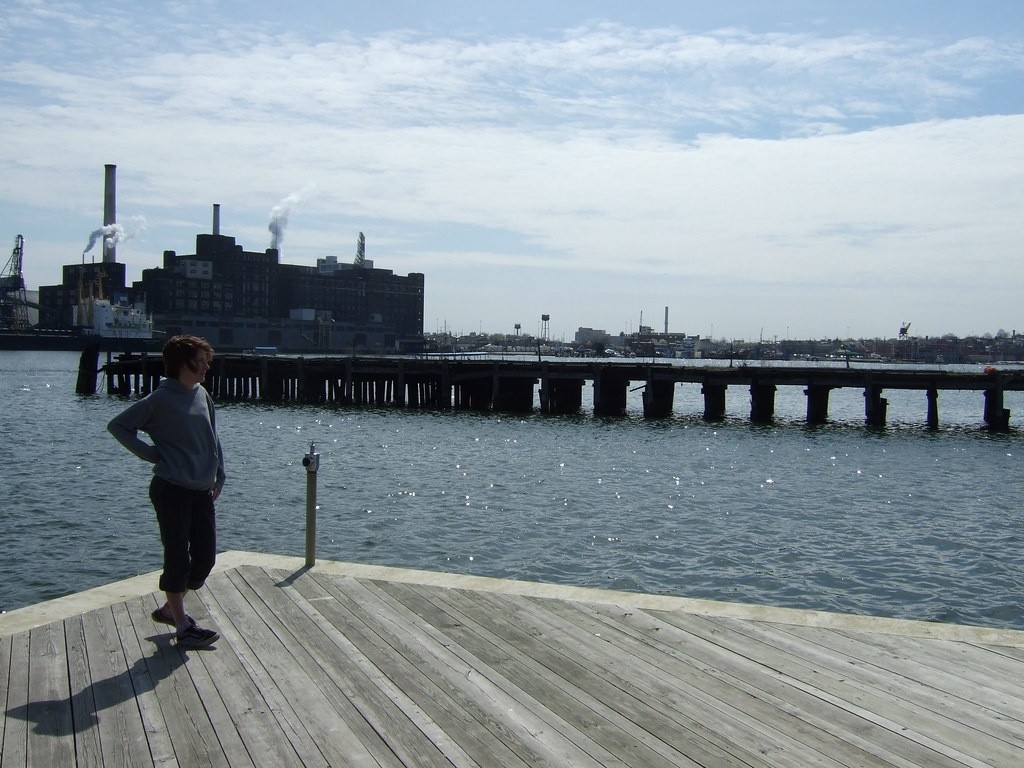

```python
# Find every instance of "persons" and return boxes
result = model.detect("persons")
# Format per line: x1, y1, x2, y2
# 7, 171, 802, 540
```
107, 332, 225, 648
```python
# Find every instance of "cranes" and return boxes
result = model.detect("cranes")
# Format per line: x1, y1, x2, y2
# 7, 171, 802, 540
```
1, 232, 35, 333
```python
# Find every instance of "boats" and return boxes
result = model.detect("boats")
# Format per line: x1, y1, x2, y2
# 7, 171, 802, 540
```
1, 330, 68, 352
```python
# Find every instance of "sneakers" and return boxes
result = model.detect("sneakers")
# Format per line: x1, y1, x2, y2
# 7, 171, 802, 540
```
177, 625, 219, 647
152, 609, 197, 627
